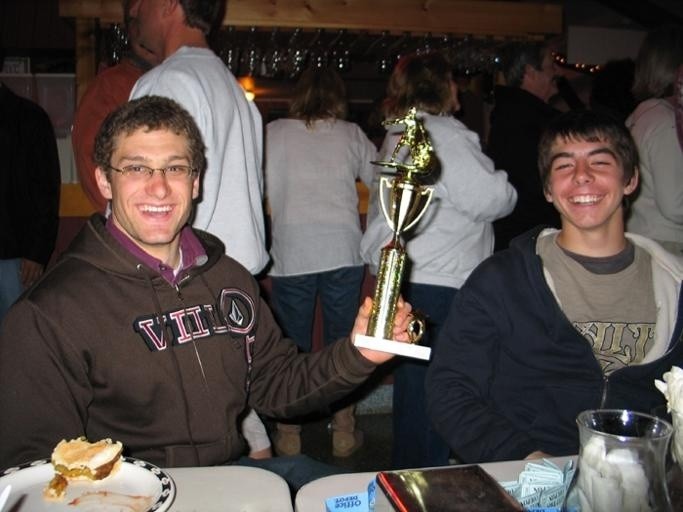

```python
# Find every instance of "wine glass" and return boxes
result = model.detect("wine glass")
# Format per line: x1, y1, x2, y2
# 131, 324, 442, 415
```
108, 21, 509, 84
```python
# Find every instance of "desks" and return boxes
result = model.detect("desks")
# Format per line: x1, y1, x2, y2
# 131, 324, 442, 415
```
160, 464, 294, 512
296, 455, 580, 512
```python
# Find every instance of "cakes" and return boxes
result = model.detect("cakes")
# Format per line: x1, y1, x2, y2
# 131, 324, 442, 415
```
50, 435, 124, 481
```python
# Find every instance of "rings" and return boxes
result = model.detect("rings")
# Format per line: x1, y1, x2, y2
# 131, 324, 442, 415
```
407, 314, 424, 343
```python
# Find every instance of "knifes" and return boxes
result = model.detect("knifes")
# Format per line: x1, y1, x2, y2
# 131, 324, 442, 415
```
0, 484, 12, 511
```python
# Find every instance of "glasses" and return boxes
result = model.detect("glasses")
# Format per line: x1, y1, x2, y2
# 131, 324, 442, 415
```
105, 166, 200, 181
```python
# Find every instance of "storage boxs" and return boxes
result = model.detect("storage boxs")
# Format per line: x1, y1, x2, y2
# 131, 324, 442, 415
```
0, 71, 37, 105
34, 70, 77, 138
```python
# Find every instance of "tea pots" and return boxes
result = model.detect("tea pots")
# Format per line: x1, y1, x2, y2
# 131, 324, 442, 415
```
574, 408, 674, 512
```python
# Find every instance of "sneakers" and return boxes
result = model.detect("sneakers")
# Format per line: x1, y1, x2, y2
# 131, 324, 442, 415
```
332, 403, 357, 457
269, 423, 302, 458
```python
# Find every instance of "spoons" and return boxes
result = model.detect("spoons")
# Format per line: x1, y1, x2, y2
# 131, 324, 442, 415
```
63, 480, 155, 505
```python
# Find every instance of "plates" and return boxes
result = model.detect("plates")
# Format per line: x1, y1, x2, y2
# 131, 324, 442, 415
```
0, 458, 175, 511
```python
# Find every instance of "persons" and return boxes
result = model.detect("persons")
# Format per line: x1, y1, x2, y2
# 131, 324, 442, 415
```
483, 35, 575, 256
262, 60, 386, 458
72, 4, 161, 218
622, 30, 682, 258
0, 82, 63, 317
422, 105, 682, 464
126, 1, 270, 279
358, 49, 520, 463
0, 95, 421, 486
